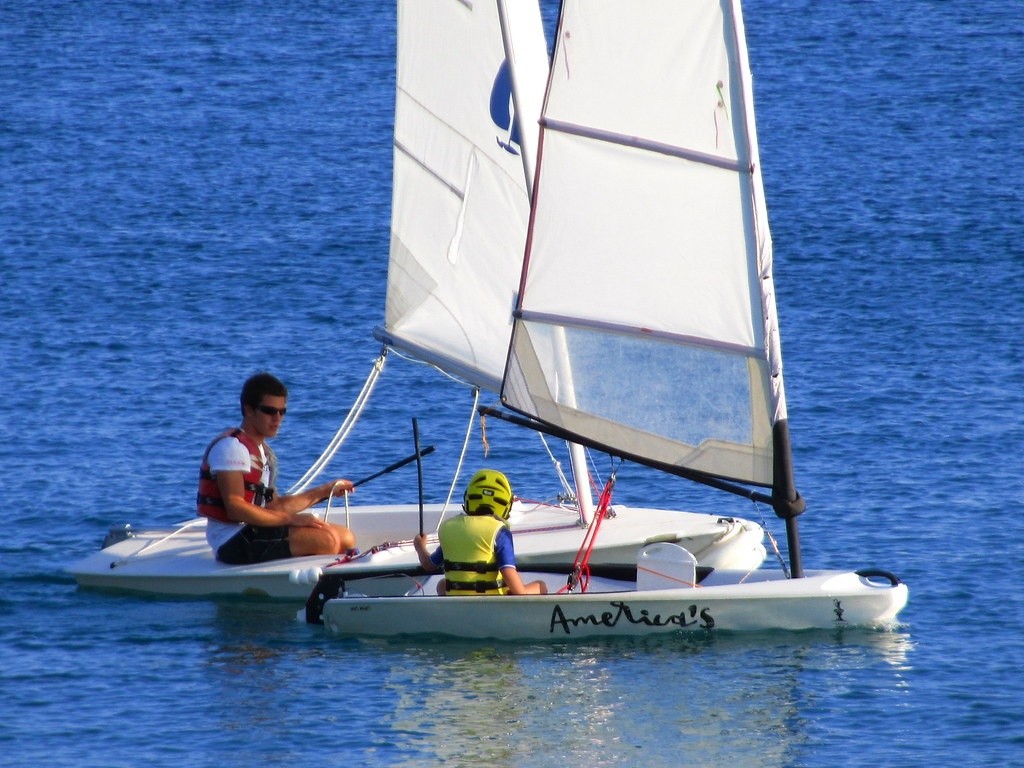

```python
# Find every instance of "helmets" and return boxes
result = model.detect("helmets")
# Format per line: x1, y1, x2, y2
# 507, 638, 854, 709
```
462, 469, 514, 522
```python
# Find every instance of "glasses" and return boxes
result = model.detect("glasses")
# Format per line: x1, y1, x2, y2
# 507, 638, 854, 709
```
250, 405, 287, 416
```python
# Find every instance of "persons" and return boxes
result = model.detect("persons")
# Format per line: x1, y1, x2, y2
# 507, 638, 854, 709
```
412, 468, 549, 596
196, 373, 354, 566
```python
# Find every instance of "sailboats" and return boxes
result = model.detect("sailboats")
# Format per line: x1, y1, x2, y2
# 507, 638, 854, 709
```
63, 1, 768, 595
324, 1, 909, 636
